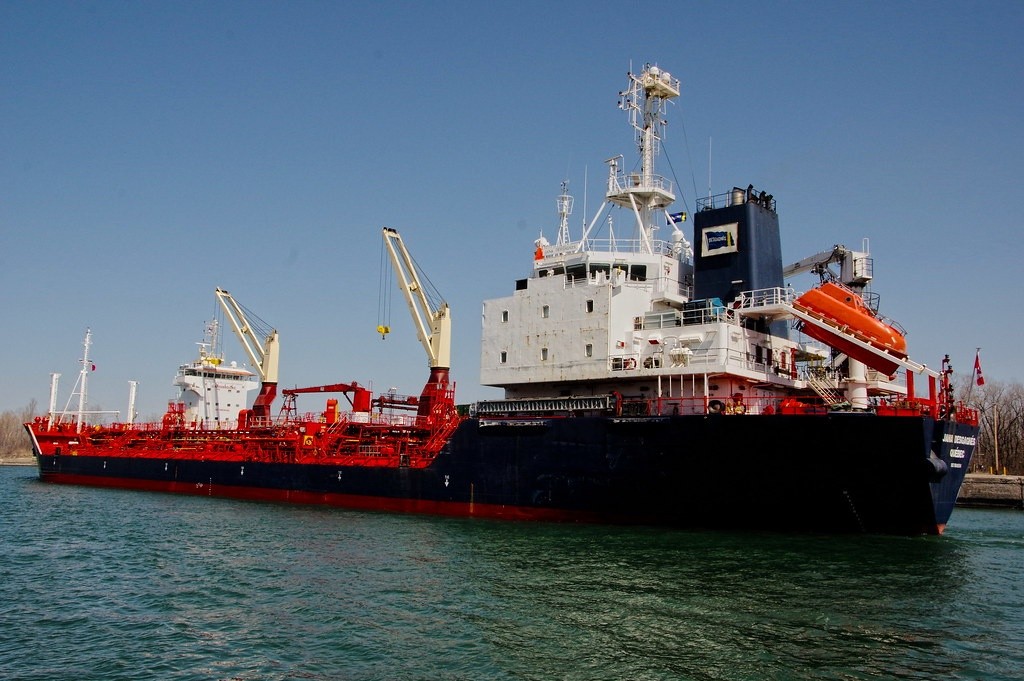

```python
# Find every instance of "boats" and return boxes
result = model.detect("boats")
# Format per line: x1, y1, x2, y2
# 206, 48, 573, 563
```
792, 283, 908, 375
669, 348, 693, 359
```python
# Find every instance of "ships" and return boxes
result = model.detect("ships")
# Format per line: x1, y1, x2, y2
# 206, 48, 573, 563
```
21, 59, 978, 542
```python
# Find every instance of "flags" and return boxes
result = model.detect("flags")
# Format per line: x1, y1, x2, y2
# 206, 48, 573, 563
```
92, 364, 96, 371
975, 352, 985, 386
666, 211, 686, 225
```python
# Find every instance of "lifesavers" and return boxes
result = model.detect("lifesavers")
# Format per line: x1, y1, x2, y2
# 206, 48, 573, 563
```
733, 402, 746, 415
625, 357, 637, 369
708, 400, 724, 414
